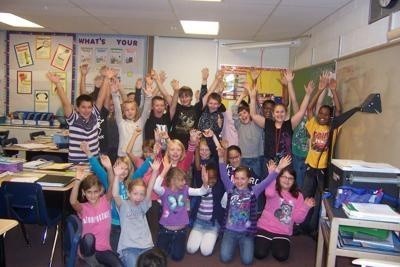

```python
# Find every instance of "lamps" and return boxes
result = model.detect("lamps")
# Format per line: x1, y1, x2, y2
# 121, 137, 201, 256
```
223, 39, 297, 50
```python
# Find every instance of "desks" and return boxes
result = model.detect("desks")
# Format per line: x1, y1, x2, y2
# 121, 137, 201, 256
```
3, 144, 69, 163
2, 163, 91, 208
1, 218, 19, 267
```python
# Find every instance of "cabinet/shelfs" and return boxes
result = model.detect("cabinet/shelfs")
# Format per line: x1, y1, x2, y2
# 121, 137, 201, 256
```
315, 194, 400, 267
0, 123, 69, 143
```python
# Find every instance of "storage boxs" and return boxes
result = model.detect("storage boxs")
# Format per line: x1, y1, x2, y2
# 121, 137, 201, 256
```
328, 163, 400, 199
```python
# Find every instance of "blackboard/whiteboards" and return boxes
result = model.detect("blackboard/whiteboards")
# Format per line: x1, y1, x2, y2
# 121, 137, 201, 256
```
289, 37, 400, 170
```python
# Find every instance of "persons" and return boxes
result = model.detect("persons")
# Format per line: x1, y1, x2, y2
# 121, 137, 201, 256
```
48, 63, 342, 266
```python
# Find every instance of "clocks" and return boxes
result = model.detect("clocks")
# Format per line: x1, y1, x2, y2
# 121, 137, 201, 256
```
379, 0, 396, 9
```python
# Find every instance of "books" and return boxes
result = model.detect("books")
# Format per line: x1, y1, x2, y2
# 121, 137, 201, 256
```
34, 173, 76, 187
352, 231, 395, 248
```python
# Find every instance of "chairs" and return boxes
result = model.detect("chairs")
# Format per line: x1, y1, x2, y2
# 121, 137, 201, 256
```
1, 180, 62, 260
64, 214, 82, 267
30, 131, 46, 140
0, 130, 9, 145
31, 155, 63, 163
2, 137, 19, 158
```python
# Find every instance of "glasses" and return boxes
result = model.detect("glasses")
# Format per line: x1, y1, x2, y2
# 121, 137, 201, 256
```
280, 175, 295, 182
